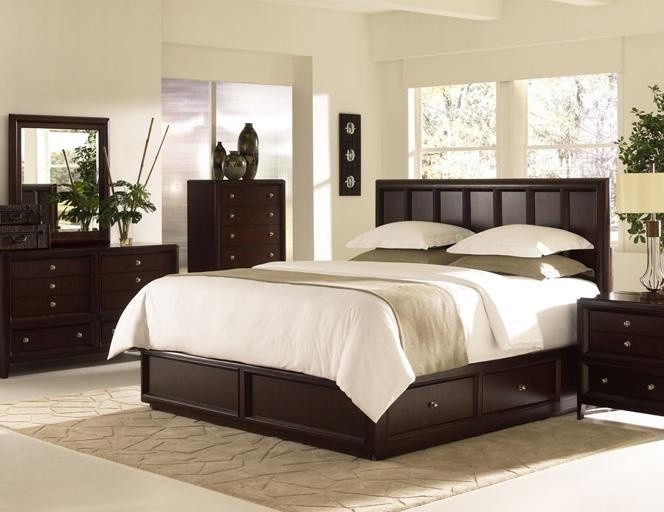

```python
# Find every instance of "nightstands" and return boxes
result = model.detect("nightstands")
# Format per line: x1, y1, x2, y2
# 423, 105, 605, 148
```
573, 289, 663, 422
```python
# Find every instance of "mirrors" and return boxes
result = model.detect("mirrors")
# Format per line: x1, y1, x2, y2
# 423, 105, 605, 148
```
7, 112, 113, 249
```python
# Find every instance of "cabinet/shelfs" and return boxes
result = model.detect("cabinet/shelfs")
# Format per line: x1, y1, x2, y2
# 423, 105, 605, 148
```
94, 242, 181, 363
185, 178, 288, 272
1, 246, 101, 381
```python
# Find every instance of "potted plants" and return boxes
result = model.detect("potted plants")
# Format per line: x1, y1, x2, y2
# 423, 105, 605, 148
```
46, 179, 100, 232
98, 178, 157, 247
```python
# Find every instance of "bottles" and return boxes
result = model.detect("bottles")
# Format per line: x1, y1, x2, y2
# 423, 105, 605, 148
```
213, 141, 226, 180
237, 123, 258, 180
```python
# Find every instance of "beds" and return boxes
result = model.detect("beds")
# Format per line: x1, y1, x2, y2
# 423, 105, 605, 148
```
104, 176, 613, 464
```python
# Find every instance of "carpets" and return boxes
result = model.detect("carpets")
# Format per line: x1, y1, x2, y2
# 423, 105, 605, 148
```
0, 384, 664, 512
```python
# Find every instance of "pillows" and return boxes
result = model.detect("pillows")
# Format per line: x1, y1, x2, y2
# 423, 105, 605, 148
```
347, 247, 468, 271
450, 253, 597, 282
444, 221, 596, 260
342, 217, 470, 254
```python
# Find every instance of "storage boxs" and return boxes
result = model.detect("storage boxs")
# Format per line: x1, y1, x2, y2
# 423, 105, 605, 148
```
0, 201, 54, 252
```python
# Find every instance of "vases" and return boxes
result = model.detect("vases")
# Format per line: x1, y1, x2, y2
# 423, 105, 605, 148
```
213, 122, 260, 181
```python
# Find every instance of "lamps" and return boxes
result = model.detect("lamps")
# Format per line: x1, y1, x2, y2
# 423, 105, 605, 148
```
613, 172, 663, 295
